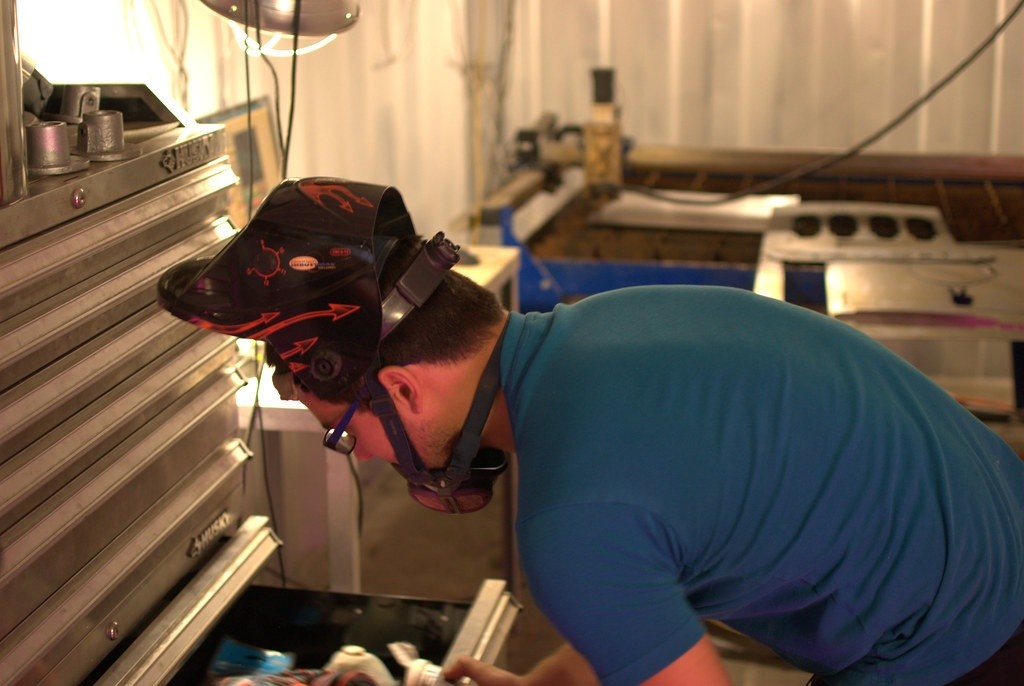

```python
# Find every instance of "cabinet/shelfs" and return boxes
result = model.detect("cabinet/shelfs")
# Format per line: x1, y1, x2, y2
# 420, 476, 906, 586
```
0, 121, 526, 686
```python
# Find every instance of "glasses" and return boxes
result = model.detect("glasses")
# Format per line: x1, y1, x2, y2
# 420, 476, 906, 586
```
322, 400, 364, 455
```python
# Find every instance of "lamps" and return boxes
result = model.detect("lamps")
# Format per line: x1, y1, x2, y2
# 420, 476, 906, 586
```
195, 0, 361, 36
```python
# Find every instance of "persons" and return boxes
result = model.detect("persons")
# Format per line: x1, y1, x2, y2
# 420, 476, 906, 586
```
157, 182, 1024, 686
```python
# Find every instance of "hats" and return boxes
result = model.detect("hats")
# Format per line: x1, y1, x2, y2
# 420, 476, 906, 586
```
155, 177, 415, 406
